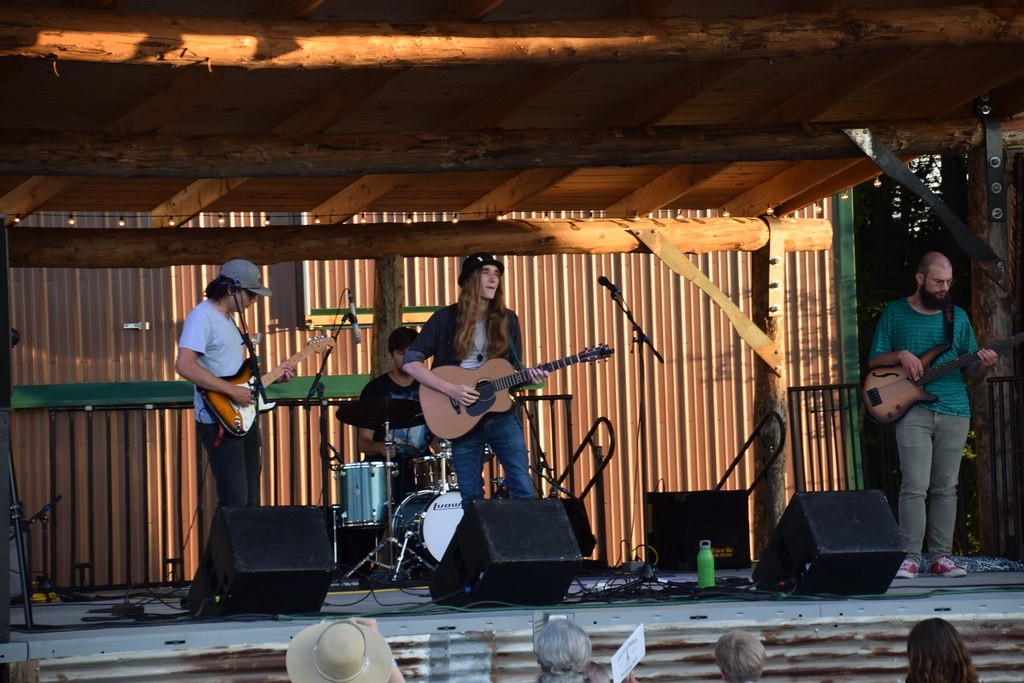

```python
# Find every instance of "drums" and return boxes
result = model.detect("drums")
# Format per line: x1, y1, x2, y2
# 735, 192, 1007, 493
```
439, 439, 495, 468
335, 461, 398, 530
414, 453, 454, 489
392, 489, 464, 580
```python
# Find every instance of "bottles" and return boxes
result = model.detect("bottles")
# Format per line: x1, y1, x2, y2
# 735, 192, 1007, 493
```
697, 540, 715, 590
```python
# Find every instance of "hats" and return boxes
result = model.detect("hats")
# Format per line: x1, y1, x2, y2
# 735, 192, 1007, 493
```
285, 621, 393, 683
218, 259, 272, 297
458, 253, 505, 288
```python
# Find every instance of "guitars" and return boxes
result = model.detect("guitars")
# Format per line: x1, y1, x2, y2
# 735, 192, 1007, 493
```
861, 331, 1024, 424
200, 335, 335, 438
419, 342, 615, 445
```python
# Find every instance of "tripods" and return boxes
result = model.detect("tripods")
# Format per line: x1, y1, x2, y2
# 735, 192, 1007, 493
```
8, 495, 94, 601
600, 292, 702, 597
340, 412, 437, 584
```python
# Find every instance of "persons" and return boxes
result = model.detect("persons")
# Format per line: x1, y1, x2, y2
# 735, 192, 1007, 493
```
285, 618, 408, 683
713, 629, 767, 683
534, 620, 593, 683
173, 260, 298, 507
357, 326, 443, 504
866, 250, 999, 580
402, 254, 552, 506
904, 618, 979, 683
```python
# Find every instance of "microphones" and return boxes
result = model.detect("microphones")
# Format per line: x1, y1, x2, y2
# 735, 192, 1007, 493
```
598, 276, 622, 295
348, 290, 361, 344
329, 444, 344, 464
217, 275, 240, 284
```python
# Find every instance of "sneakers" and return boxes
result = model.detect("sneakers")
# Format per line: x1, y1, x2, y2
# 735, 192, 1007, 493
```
895, 560, 919, 579
931, 555, 967, 578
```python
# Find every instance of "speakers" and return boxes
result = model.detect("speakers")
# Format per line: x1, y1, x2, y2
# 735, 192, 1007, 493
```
753, 489, 907, 597
431, 499, 583, 610
644, 489, 750, 573
8, 521, 30, 604
185, 505, 337, 619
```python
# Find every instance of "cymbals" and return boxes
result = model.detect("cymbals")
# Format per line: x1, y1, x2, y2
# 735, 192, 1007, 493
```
335, 398, 426, 430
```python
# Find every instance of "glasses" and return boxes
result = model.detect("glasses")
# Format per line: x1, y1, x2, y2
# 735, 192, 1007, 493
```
239, 287, 254, 299
922, 274, 956, 287
